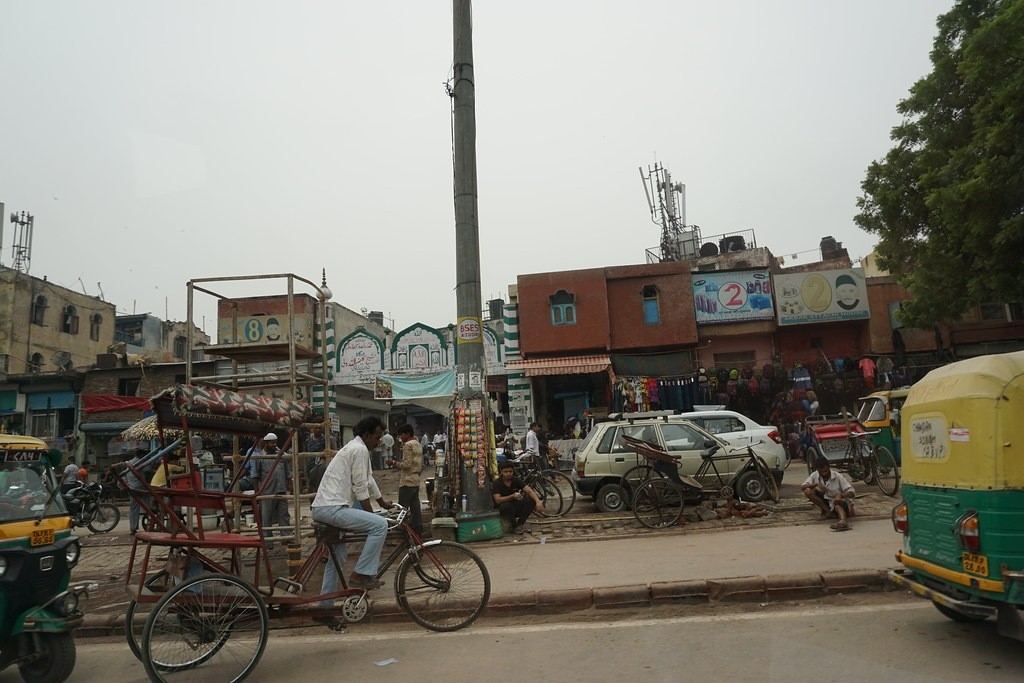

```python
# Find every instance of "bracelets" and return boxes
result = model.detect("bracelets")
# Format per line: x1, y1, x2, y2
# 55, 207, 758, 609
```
393, 460, 395, 465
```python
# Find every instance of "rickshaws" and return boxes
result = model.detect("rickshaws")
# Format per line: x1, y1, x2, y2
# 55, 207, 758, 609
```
109, 383, 492, 683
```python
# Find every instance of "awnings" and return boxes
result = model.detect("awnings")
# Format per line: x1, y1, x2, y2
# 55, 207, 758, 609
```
505, 354, 616, 385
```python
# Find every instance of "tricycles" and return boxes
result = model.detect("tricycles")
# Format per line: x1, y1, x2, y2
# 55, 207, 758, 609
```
618, 433, 779, 527
804, 412, 899, 497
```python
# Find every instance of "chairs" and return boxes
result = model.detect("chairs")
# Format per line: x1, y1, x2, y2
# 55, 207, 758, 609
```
642, 429, 652, 441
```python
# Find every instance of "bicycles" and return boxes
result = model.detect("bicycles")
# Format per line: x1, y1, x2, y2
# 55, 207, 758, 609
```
423, 438, 577, 520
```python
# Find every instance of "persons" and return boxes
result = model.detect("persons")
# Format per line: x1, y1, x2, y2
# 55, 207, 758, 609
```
101, 464, 117, 503
0, 455, 90, 513
309, 415, 395, 631
801, 457, 855, 530
492, 461, 545, 533
305, 422, 446, 511
111, 448, 185, 535
217, 433, 295, 550
525, 421, 561, 482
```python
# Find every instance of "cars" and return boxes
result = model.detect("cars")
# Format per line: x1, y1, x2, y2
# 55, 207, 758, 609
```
616, 411, 788, 485
571, 411, 784, 519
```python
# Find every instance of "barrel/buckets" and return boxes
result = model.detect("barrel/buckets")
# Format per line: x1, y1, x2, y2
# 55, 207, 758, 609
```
245, 514, 254, 526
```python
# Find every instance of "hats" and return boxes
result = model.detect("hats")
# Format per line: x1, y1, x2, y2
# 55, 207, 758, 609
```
264, 433, 277, 440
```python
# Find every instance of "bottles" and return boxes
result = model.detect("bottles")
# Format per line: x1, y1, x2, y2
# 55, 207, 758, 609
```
462, 495, 468, 513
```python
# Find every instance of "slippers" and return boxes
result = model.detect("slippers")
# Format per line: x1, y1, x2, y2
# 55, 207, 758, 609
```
830, 523, 852, 530
520, 524, 531, 533
817, 511, 837, 521
510, 526, 524, 535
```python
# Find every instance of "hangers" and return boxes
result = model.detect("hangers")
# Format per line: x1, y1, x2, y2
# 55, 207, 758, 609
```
617, 375, 698, 384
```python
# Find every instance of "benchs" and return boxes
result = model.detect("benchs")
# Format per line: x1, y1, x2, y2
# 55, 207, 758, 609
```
814, 421, 864, 438
647, 444, 683, 470
134, 487, 262, 547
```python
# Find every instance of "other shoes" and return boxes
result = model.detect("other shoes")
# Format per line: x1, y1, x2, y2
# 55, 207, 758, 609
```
312, 616, 347, 628
348, 574, 385, 588
131, 531, 137, 535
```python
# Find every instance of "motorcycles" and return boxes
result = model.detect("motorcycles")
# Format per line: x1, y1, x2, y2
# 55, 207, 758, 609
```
63, 479, 121, 534
856, 387, 910, 467
888, 344, 1024, 641
0, 432, 90, 683
